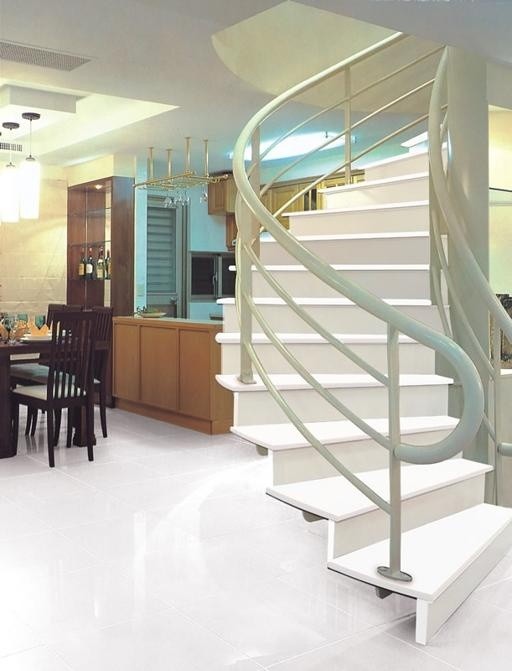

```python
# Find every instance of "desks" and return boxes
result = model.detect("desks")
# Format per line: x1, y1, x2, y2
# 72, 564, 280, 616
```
0, 341, 50, 459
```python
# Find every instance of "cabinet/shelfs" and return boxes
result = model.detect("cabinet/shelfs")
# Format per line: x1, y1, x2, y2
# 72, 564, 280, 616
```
208, 169, 364, 250
67, 176, 135, 316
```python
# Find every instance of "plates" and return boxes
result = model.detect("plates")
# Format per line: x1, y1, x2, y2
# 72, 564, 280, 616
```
19, 336, 52, 342
138, 312, 168, 319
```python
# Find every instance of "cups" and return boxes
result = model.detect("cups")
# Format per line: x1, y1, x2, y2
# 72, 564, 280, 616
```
35, 315, 46, 330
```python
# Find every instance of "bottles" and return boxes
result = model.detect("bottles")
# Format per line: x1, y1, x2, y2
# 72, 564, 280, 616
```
78, 247, 112, 281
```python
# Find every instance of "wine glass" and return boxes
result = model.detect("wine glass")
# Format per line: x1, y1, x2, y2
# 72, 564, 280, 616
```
163, 182, 210, 208
4, 315, 17, 345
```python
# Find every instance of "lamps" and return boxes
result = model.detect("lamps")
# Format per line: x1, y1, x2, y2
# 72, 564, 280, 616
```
0, 112, 40, 225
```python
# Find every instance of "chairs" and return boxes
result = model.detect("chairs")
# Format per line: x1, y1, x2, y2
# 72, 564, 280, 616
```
8, 303, 114, 468
